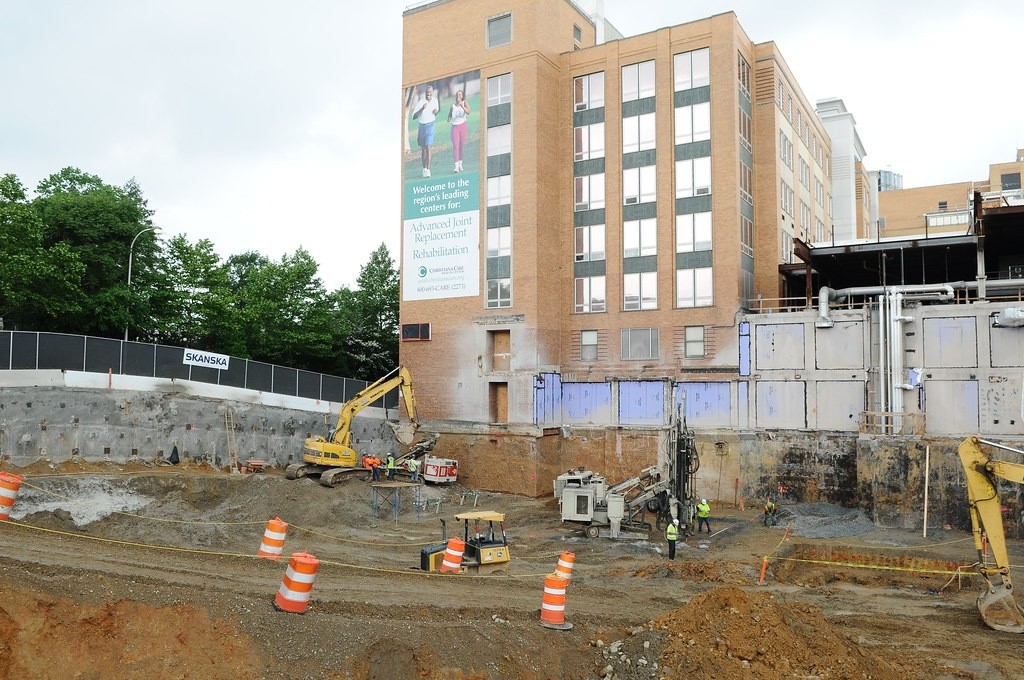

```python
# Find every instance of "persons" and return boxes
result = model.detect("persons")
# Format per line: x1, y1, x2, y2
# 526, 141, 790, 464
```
412, 84, 471, 178
362, 452, 380, 482
667, 518, 680, 561
683, 500, 697, 536
386, 453, 395, 481
409, 454, 420, 483
763, 502, 777, 526
696, 499, 712, 534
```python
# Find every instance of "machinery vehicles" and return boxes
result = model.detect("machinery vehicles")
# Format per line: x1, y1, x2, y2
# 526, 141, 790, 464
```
553, 381, 700, 539
957, 437, 1024, 634
420, 512, 511, 574
285, 362, 421, 488
394, 433, 458, 485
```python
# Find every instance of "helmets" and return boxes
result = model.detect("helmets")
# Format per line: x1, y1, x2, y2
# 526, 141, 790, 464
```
387, 452, 391, 456
673, 518, 679, 526
367, 454, 371, 456
701, 498, 706, 505
362, 451, 367, 455
372, 454, 375, 458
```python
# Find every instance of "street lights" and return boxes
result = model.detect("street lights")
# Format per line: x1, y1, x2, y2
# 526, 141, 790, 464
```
123, 226, 162, 375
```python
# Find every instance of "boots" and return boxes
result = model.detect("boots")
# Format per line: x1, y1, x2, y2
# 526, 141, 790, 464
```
458, 159, 464, 171
453, 161, 460, 174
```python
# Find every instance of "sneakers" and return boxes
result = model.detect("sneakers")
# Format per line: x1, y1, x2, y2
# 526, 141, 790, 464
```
422, 167, 432, 178
